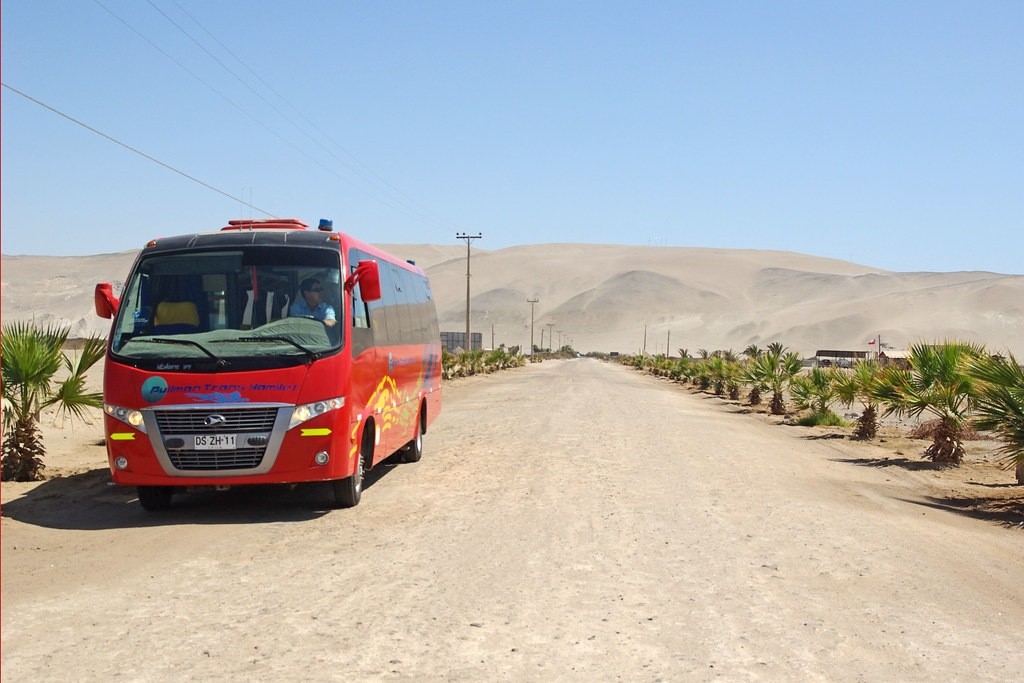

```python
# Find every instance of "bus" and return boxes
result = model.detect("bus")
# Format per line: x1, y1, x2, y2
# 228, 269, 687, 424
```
95, 217, 443, 513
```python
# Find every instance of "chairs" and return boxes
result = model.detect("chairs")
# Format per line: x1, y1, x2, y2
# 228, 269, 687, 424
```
153, 301, 200, 335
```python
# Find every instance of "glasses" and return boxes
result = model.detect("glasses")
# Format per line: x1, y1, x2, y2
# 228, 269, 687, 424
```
309, 287, 324, 293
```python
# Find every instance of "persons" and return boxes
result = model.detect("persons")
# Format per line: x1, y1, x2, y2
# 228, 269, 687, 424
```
289, 278, 337, 328
